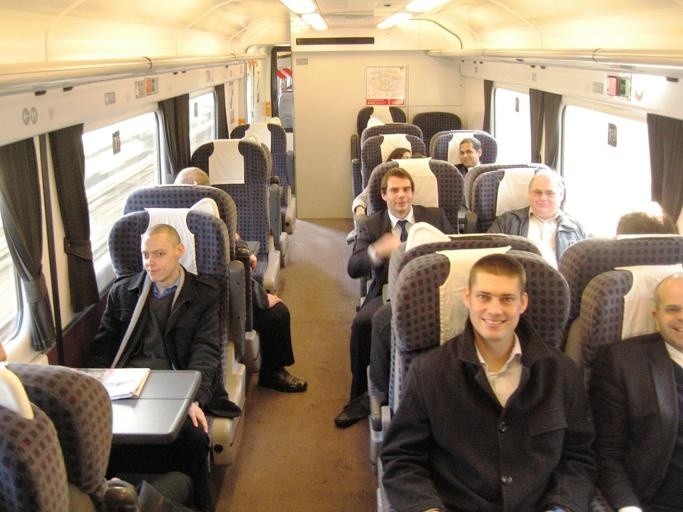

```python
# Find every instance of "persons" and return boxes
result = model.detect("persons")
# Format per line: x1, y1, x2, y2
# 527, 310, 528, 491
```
384, 254, 595, 510
170, 166, 307, 393
616, 200, 677, 234
485, 167, 584, 270
453, 138, 482, 179
334, 169, 455, 428
229, 231, 259, 268
592, 273, 682, 511
80, 224, 223, 446
405, 153, 428, 160
348, 149, 414, 308
99, 461, 191, 510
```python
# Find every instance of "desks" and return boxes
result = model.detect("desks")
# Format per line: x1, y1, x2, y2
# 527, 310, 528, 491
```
69, 368, 203, 447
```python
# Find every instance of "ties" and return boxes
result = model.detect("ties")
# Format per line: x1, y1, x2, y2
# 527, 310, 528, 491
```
398, 220, 410, 243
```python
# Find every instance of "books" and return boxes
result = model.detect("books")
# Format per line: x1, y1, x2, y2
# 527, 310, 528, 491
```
99, 367, 150, 400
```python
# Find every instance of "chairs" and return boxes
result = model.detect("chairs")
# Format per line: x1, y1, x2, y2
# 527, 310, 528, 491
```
365, 233, 682, 511
231, 120, 294, 233
0, 361, 139, 510
109, 207, 247, 467
350, 106, 569, 313
125, 184, 262, 372
192, 138, 282, 298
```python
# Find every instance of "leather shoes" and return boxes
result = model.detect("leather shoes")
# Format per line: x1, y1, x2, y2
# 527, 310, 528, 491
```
258, 367, 307, 392
335, 399, 368, 425
